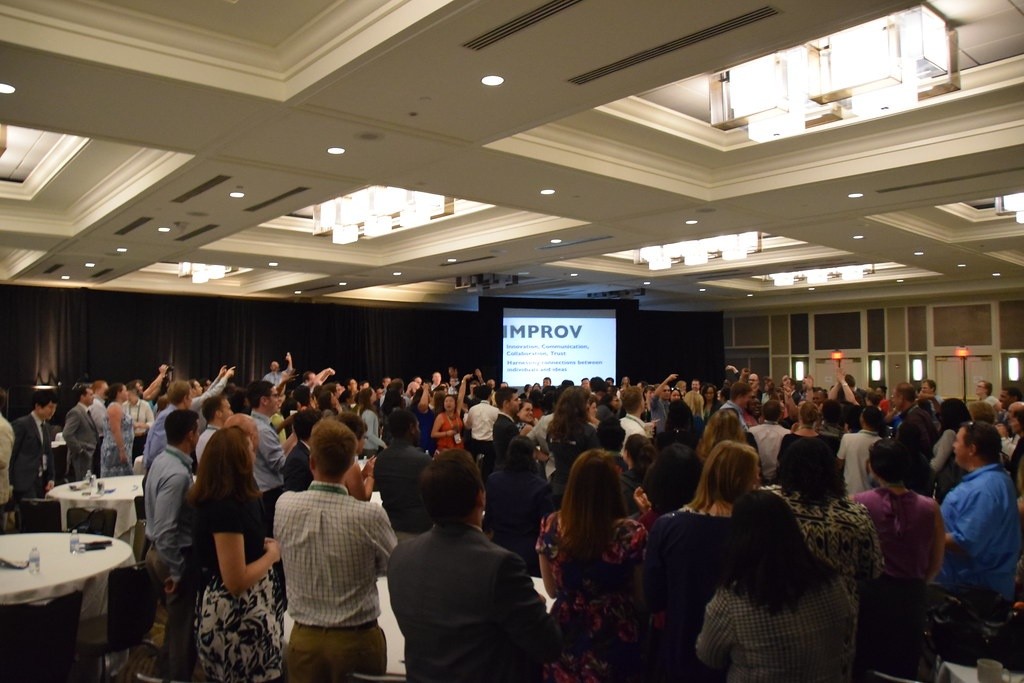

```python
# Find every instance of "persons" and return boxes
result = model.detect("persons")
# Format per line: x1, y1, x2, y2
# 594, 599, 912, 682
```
0, 352, 1024, 682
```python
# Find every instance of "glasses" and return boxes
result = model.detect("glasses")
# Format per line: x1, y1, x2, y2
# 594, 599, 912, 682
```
670, 387, 680, 391
967, 421, 974, 430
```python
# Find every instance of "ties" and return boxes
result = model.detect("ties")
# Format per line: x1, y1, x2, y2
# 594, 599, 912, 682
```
41, 423, 48, 472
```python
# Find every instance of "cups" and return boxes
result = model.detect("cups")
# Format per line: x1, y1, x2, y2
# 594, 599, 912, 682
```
976, 659, 1012, 683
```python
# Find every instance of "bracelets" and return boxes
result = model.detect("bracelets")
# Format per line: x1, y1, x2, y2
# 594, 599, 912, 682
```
843, 383, 848, 386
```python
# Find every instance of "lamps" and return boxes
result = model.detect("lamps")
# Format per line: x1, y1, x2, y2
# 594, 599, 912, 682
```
830, 349, 843, 369
995, 192, 1024, 226
178, 260, 232, 285
955, 346, 970, 405
308, 179, 457, 247
708, 1, 949, 146
768, 263, 871, 285
632, 231, 761, 270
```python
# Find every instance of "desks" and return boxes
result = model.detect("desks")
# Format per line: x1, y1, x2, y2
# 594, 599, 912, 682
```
0, 454, 558, 683
935, 661, 1024, 683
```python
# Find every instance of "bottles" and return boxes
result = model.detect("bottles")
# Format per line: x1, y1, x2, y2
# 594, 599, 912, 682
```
68, 529, 81, 558
84, 470, 104, 497
28, 547, 40, 576
